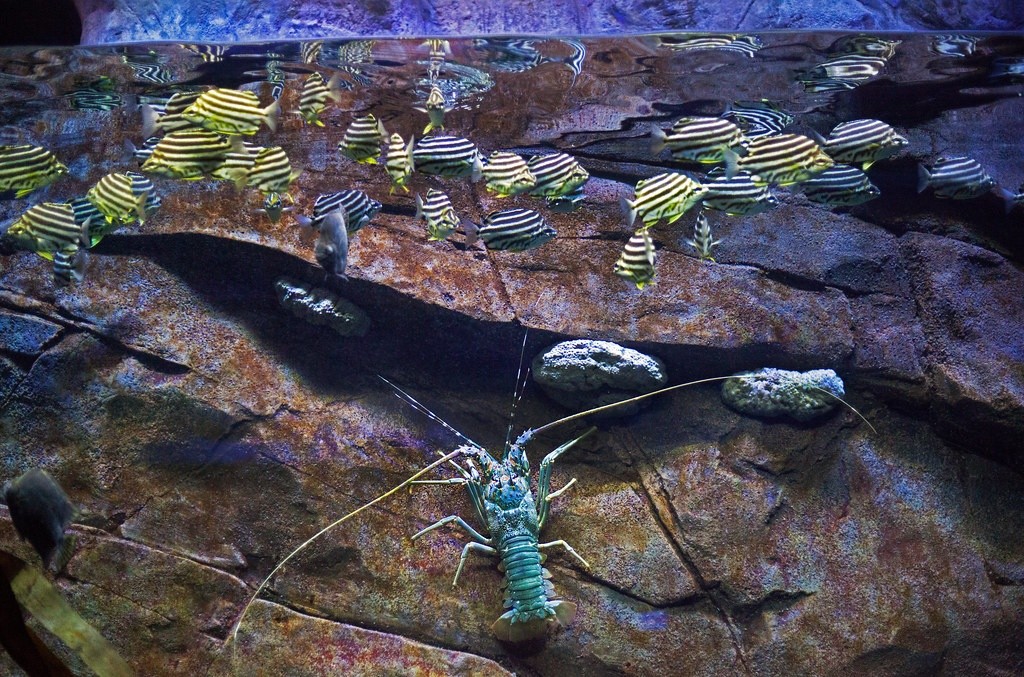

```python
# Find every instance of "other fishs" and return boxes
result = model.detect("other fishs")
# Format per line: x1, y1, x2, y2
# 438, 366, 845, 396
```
0, 34, 1020, 293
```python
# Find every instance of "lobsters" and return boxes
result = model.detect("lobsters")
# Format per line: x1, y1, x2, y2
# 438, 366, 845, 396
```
230, 281, 878, 672
